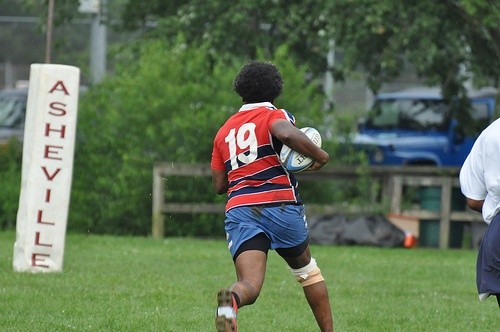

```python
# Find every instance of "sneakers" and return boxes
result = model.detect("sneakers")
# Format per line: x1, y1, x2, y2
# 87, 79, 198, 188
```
215, 289, 236, 332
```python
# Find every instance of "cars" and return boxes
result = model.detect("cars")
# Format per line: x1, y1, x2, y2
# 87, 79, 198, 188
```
0, 87, 28, 138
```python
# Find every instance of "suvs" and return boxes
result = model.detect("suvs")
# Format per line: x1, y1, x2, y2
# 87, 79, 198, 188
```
350, 87, 495, 166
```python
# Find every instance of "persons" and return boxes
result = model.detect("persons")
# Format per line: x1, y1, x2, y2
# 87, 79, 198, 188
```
459, 117, 500, 308
211, 62, 333, 332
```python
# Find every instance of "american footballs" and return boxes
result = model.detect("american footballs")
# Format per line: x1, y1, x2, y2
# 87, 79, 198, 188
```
280, 126, 322, 173
